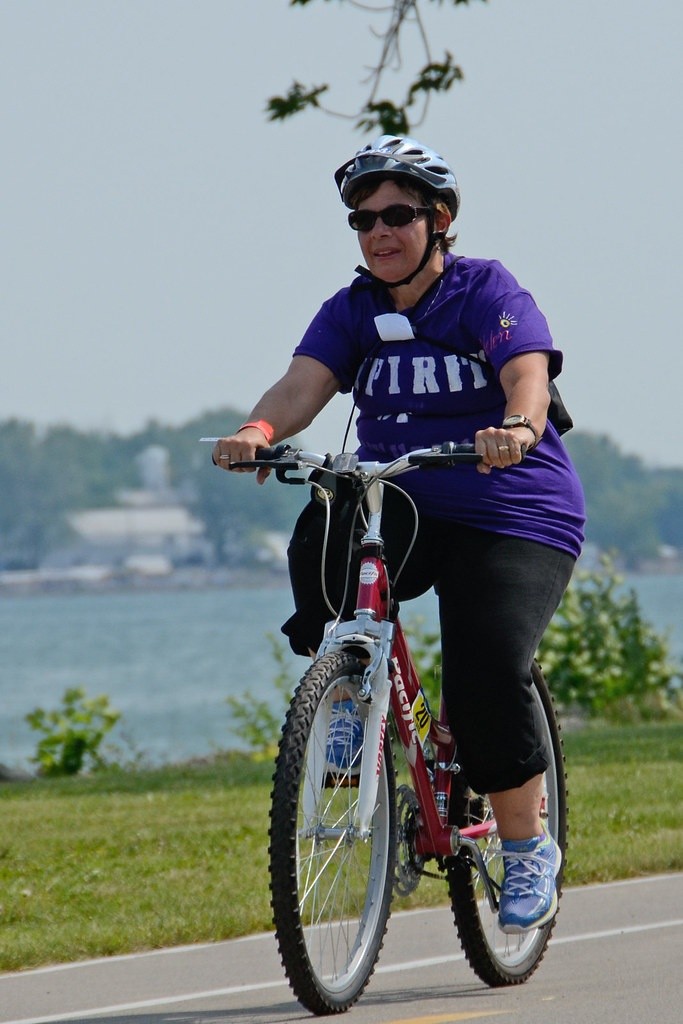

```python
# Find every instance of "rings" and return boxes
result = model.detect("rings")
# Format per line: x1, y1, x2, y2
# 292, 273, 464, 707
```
230, 461, 236, 464
499, 446, 509, 450
220, 455, 229, 459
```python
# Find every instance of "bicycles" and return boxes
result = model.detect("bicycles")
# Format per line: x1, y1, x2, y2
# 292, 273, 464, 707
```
198, 435, 570, 1015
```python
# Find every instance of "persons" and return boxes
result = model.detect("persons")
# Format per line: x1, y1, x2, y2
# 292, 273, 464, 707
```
211, 134, 584, 934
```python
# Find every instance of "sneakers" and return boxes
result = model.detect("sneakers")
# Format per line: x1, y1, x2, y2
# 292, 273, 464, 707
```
497, 819, 562, 934
325, 700, 365, 775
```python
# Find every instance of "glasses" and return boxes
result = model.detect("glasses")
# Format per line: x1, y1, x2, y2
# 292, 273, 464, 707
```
348, 204, 432, 231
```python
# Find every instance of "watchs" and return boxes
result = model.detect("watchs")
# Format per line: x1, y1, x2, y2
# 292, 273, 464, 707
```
503, 414, 539, 451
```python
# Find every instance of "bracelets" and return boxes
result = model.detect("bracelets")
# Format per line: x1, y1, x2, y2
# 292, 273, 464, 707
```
237, 417, 275, 444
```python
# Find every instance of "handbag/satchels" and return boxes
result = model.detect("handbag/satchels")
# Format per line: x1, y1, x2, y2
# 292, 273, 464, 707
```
543, 376, 574, 438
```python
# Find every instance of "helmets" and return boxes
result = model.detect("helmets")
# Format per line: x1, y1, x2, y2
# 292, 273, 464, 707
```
333, 134, 460, 222
